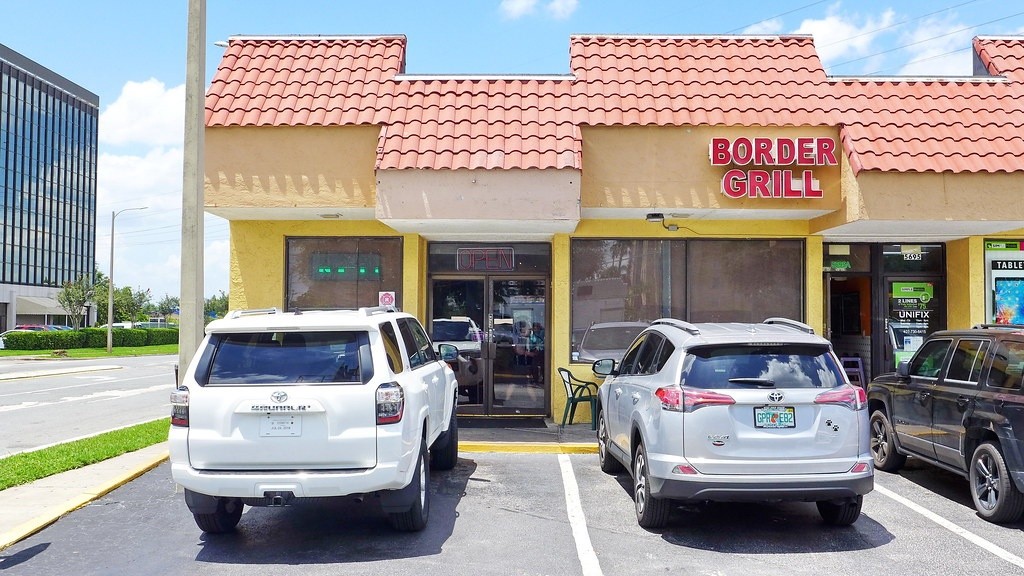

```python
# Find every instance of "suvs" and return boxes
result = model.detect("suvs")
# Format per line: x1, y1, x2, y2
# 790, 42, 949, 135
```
428, 314, 486, 405
572, 319, 655, 366
168, 303, 461, 535
592, 316, 876, 531
865, 322, 1024, 525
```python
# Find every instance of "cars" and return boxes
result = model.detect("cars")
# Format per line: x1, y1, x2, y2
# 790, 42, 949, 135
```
0, 318, 178, 349
492, 312, 516, 346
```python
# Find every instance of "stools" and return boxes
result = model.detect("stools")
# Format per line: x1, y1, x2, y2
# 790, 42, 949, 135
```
840, 357, 866, 391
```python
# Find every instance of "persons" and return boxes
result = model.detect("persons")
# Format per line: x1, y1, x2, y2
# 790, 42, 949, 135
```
517, 320, 545, 390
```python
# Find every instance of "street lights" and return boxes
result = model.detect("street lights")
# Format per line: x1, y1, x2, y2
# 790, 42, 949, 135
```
107, 205, 150, 354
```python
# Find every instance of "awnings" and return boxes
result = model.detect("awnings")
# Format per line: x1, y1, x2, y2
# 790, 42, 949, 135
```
16, 295, 88, 328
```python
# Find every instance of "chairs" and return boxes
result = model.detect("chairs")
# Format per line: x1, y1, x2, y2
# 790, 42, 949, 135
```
338, 342, 358, 366
281, 334, 306, 355
557, 366, 599, 431
934, 347, 970, 380
254, 339, 283, 372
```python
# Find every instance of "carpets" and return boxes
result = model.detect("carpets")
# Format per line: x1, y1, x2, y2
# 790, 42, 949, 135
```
457, 417, 548, 428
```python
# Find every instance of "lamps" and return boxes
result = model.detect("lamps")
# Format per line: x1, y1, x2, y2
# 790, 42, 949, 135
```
645, 213, 664, 223
